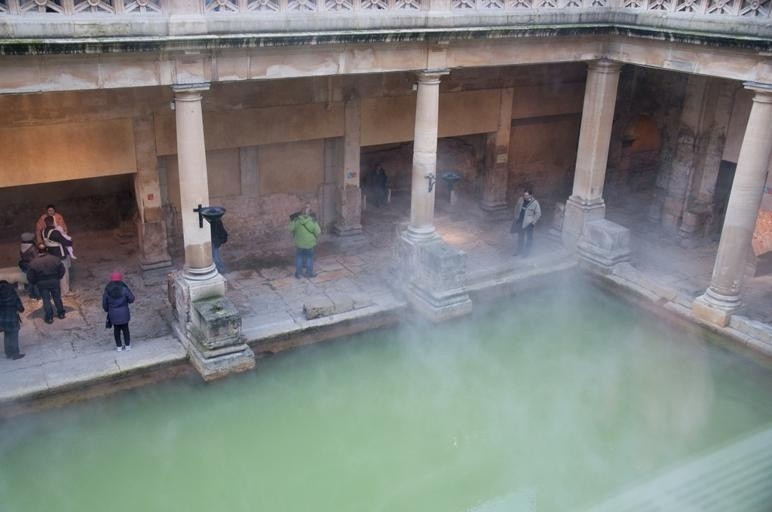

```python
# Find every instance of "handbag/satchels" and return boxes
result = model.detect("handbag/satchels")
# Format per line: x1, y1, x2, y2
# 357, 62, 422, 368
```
511, 221, 519, 235
106, 314, 110, 329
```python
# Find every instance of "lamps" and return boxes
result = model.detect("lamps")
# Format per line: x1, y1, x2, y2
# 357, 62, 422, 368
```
425, 173, 461, 192
193, 204, 225, 227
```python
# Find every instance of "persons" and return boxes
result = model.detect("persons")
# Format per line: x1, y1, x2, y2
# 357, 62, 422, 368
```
0, 279, 27, 361
40, 215, 72, 296
35, 205, 67, 250
370, 164, 389, 209
19, 232, 41, 298
511, 186, 542, 259
27, 243, 67, 323
211, 220, 228, 274
57, 226, 77, 259
288, 206, 321, 279
102, 271, 136, 351
290, 202, 317, 221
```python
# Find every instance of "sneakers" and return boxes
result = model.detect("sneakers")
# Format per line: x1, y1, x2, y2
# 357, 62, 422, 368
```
306, 272, 316, 279
29, 292, 73, 301
512, 248, 521, 255
117, 346, 122, 351
47, 317, 53, 323
5, 353, 12, 359
59, 313, 66, 318
125, 346, 130, 350
13, 353, 25, 360
295, 273, 303, 279
521, 253, 530, 258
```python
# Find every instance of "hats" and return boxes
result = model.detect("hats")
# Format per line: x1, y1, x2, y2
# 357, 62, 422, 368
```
21, 233, 35, 242
111, 272, 122, 281
